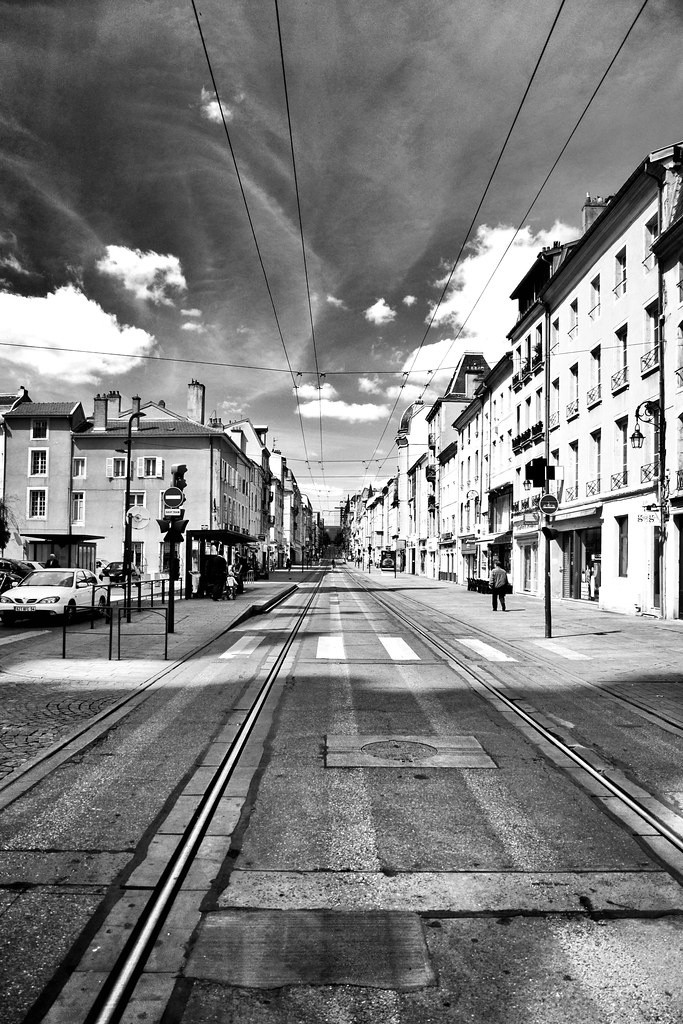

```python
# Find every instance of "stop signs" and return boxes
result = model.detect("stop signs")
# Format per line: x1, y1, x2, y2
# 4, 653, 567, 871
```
163, 487, 184, 510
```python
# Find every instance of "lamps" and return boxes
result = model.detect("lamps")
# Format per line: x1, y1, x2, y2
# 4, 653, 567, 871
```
630, 401, 662, 448
464, 490, 480, 513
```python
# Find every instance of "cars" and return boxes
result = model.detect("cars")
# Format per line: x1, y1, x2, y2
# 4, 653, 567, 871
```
98, 562, 141, 588
94, 558, 112, 579
0, 568, 111, 620
0, 556, 34, 583
0, 570, 23, 596
17, 559, 48, 575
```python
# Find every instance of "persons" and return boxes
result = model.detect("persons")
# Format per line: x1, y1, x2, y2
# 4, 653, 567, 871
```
331, 558, 335, 569
376, 559, 380, 568
231, 557, 241, 584
45, 553, 61, 568
209, 550, 228, 602
235, 552, 246, 595
270, 559, 276, 573
288, 560, 292, 573
488, 561, 508, 611
234, 566, 239, 595
225, 571, 239, 600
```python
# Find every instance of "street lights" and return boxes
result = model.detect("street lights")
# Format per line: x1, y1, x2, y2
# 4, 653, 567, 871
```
466, 489, 487, 579
120, 412, 152, 625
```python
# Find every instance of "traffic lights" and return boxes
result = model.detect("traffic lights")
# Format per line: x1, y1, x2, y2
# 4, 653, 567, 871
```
156, 518, 169, 533
176, 519, 189, 544
